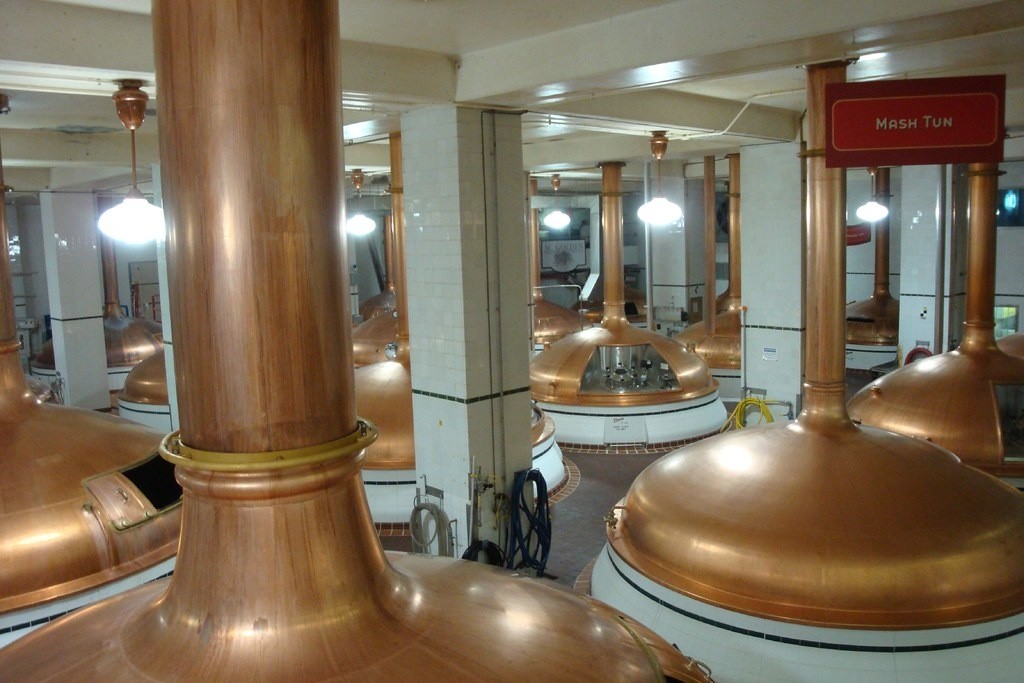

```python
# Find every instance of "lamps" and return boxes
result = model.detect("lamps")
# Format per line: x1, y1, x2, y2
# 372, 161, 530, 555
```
857, 166, 889, 222
637, 131, 682, 227
97, 81, 166, 245
544, 174, 571, 230
345, 169, 376, 237
